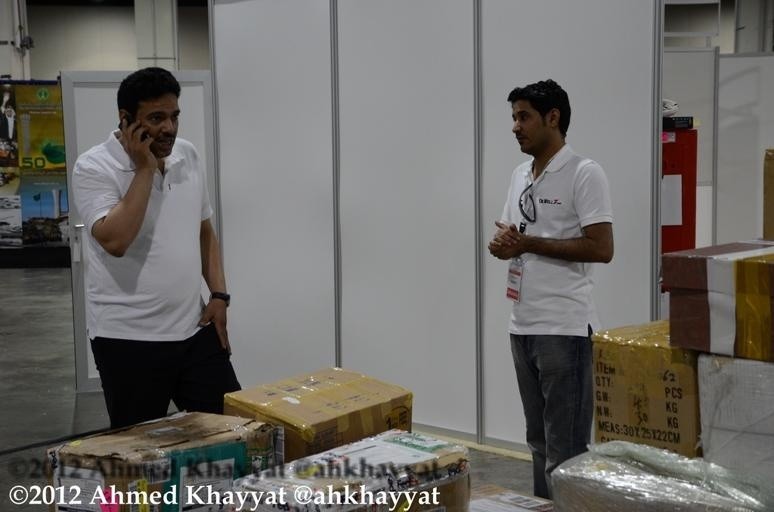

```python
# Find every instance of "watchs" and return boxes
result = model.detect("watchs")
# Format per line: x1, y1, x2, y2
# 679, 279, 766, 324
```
209, 291, 232, 307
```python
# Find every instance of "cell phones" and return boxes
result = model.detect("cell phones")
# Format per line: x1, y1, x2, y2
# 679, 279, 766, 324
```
117, 115, 148, 146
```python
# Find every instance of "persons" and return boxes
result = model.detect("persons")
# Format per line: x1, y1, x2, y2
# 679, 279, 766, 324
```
69, 67, 244, 429
484, 79, 615, 498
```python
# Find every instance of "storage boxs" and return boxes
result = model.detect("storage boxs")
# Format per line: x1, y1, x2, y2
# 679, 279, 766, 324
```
46, 148, 774, 512
